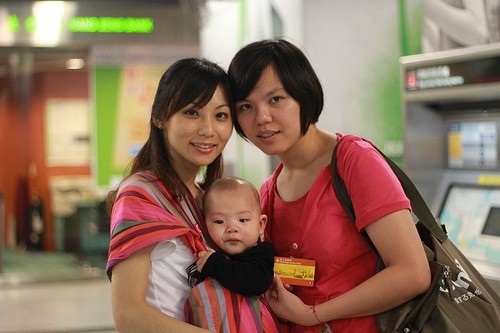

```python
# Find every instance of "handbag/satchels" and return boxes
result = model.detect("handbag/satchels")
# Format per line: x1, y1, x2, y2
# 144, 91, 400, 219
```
375, 217, 500, 333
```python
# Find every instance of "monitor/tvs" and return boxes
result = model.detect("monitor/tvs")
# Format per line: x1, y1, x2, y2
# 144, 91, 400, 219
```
437, 183, 500, 281
